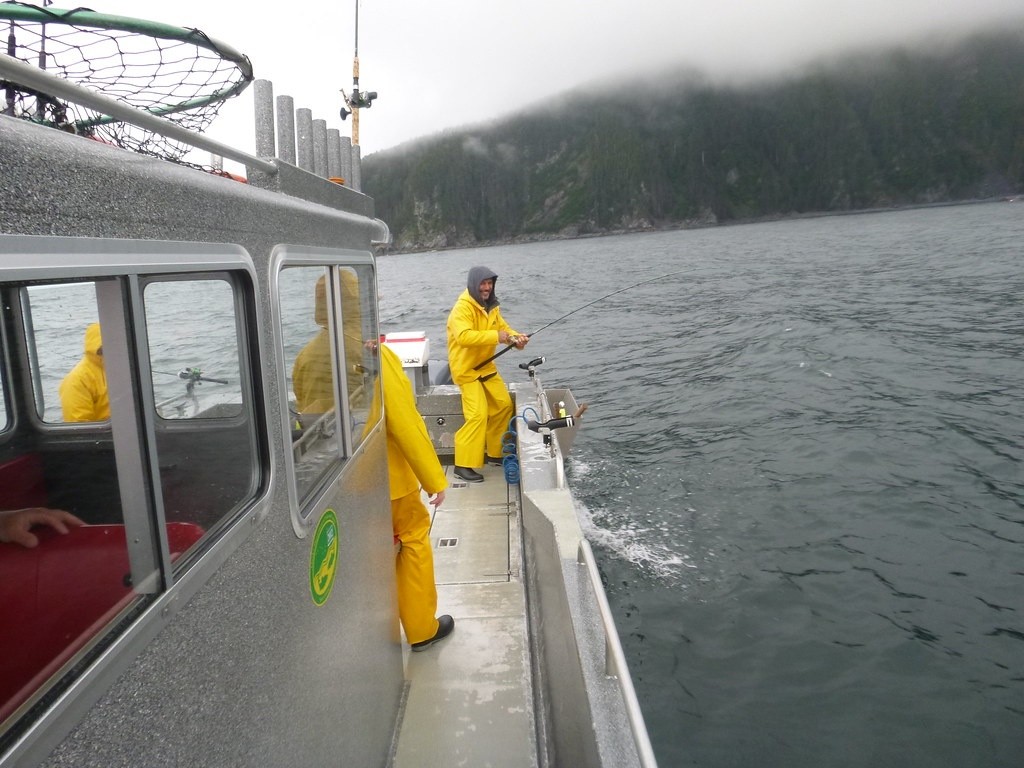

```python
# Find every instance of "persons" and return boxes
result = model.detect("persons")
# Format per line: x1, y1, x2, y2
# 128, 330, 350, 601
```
290, 266, 529, 651
55, 323, 111, 426
0, 507, 86, 548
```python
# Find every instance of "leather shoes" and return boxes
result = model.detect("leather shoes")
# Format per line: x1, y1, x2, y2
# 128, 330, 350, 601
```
410, 615, 455, 652
453, 466, 484, 482
487, 455, 504, 466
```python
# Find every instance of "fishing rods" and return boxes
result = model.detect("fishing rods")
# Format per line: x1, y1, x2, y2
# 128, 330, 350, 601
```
52, 351, 228, 383
338, 0, 379, 145
475, 263, 721, 371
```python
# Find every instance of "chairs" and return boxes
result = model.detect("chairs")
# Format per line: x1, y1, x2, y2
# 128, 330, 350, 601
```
0, 452, 205, 709
